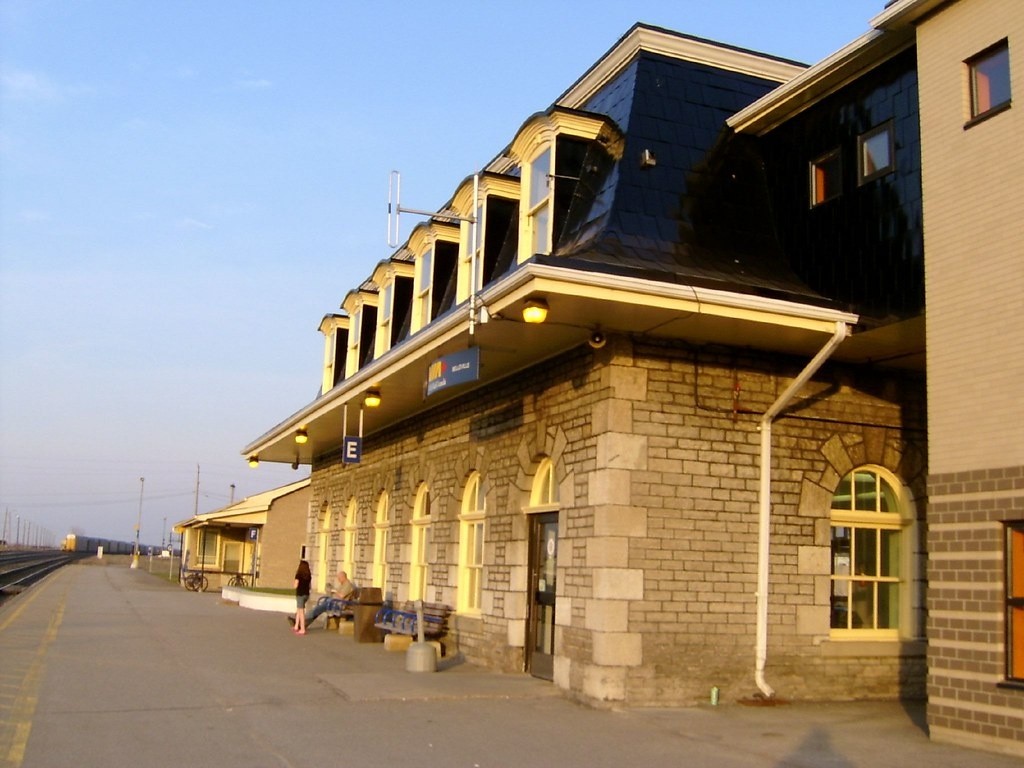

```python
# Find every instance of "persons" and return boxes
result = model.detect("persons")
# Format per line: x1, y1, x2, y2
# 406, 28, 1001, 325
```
287, 571, 354, 629
292, 560, 312, 635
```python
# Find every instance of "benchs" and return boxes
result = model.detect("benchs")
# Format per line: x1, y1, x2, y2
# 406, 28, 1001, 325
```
317, 596, 354, 636
374, 600, 453, 659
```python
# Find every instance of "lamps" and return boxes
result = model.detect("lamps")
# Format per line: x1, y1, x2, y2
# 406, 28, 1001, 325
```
295, 431, 307, 444
249, 456, 259, 468
521, 298, 549, 324
364, 391, 381, 407
292, 457, 299, 470
588, 332, 607, 349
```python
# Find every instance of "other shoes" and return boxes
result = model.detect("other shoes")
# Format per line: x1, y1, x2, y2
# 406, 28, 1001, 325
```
295, 631, 306, 636
290, 627, 298, 633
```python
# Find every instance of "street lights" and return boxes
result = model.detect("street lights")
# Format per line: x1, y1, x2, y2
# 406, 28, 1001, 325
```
131, 476, 145, 569
